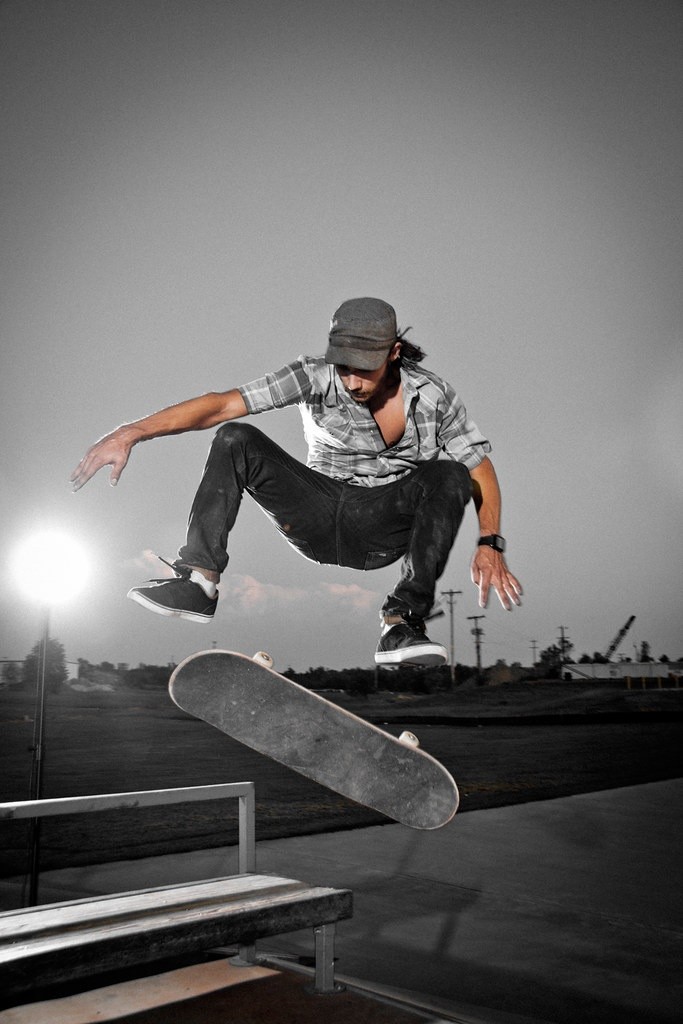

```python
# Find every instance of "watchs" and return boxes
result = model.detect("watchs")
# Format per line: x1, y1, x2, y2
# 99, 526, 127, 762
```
477, 533, 507, 553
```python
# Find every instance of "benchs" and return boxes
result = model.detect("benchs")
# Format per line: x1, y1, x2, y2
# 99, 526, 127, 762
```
0, 780, 355, 1011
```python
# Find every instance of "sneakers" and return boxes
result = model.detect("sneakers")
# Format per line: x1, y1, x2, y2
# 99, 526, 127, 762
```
127, 556, 221, 624
374, 619, 447, 667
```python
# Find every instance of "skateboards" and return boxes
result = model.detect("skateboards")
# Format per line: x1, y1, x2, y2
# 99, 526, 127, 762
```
165, 647, 461, 832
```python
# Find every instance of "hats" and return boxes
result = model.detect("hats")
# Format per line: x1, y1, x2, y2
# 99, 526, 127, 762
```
324, 297, 397, 371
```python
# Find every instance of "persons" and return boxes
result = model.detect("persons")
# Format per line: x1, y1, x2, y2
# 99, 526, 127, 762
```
69, 296, 526, 668
